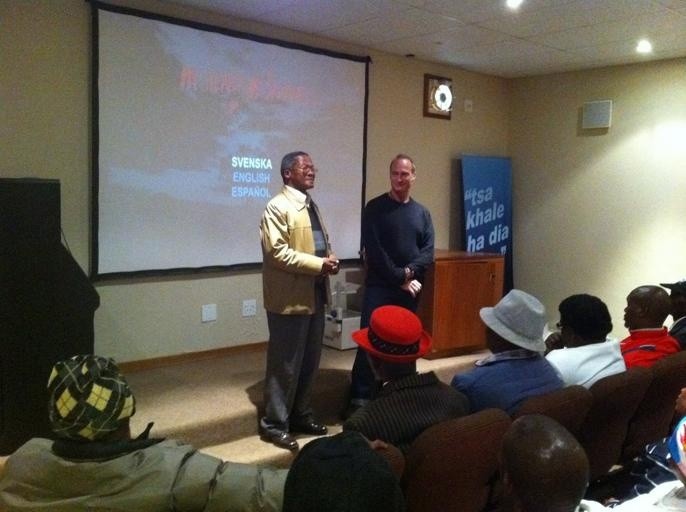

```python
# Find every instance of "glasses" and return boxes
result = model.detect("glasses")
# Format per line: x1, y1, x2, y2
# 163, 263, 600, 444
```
553, 321, 569, 327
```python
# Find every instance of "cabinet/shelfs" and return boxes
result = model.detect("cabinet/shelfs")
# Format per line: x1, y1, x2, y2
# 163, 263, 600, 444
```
424, 250, 506, 360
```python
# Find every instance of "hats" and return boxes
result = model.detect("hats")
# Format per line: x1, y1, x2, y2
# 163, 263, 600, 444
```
479, 290, 547, 355
662, 282, 685, 295
46, 354, 135, 441
351, 305, 432, 363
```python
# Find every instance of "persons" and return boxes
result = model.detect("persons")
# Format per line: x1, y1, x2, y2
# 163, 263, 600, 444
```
675, 387, 686, 415
573, 415, 686, 512
494, 413, 610, 511
660, 281, 686, 351
283, 431, 402, 511
345, 304, 469, 447
256, 152, 341, 449
543, 293, 628, 389
621, 284, 681, 369
0, 352, 406, 511
349, 153, 435, 413
450, 290, 569, 412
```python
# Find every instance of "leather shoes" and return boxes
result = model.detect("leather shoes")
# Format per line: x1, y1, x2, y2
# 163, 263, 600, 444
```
301, 422, 328, 434
270, 432, 298, 451
